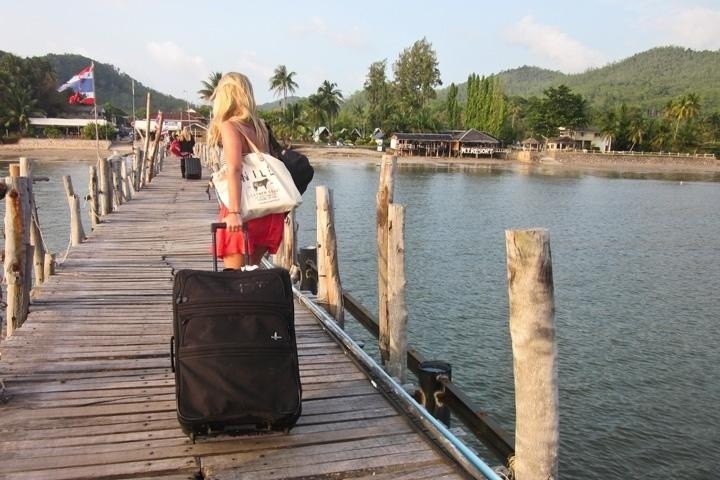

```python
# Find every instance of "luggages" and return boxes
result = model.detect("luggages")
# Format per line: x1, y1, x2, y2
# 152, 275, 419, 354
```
169, 219, 307, 436
184, 152, 202, 179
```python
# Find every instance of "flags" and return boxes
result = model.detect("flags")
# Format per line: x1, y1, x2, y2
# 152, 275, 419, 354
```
57, 66, 97, 106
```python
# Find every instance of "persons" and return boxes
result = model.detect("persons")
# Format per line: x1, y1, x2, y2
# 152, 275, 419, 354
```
169, 127, 195, 178
208, 73, 289, 271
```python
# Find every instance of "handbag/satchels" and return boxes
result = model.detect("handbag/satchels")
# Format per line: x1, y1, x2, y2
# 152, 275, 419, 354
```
206, 120, 300, 221
169, 138, 183, 156
260, 120, 315, 196
